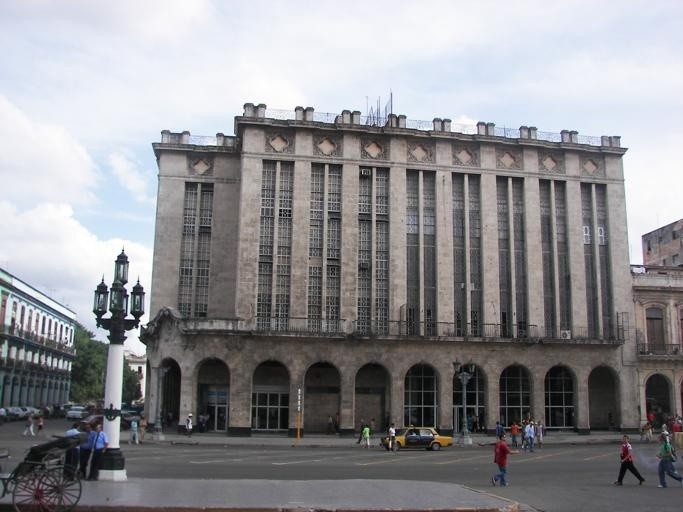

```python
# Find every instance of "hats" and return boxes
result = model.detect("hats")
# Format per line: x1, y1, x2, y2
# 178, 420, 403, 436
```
188, 413, 193, 417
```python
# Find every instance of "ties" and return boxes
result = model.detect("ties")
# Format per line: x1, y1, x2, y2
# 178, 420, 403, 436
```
92, 432, 100, 450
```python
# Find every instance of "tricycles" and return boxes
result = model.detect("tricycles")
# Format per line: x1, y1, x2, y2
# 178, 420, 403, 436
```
1, 433, 89, 512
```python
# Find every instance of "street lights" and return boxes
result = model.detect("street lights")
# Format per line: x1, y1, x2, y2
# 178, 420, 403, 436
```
91, 245, 148, 482
450, 356, 478, 446
150, 363, 174, 434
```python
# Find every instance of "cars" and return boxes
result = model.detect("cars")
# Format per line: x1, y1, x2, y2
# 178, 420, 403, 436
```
380, 424, 454, 451
0, 401, 141, 432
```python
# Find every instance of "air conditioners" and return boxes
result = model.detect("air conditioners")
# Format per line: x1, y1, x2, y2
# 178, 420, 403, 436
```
560, 329, 572, 339
360, 262, 368, 269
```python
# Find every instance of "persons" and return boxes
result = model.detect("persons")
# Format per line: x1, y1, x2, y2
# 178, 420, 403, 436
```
23, 410, 149, 481
655, 431, 683, 488
388, 422, 397, 453
197, 411, 210, 433
492, 432, 520, 487
408, 429, 417, 435
640, 409, 683, 444
183, 413, 193, 440
468, 413, 545, 453
326, 412, 376, 449
613, 434, 645, 486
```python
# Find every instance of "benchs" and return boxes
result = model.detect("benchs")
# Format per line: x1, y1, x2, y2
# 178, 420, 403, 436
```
12, 432, 89, 476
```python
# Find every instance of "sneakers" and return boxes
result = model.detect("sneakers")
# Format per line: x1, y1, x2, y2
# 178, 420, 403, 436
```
657, 484, 666, 489
492, 476, 496, 486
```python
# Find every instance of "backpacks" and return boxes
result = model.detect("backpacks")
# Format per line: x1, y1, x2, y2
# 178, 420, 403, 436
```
664, 440, 677, 462
